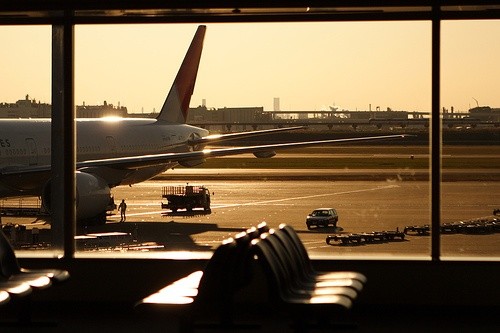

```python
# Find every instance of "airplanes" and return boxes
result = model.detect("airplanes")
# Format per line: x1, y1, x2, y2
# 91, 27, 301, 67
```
0, 24, 424, 231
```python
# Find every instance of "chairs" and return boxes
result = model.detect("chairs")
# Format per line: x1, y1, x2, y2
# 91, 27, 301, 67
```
0, 228, 70, 317
133, 222, 366, 333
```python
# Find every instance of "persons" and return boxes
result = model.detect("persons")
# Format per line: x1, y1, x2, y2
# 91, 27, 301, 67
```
118, 199, 127, 221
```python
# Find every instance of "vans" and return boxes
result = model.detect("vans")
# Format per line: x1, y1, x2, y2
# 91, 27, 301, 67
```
306, 208, 340, 229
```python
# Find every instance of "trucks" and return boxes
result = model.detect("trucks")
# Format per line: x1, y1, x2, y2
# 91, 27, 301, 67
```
160, 182, 216, 216
